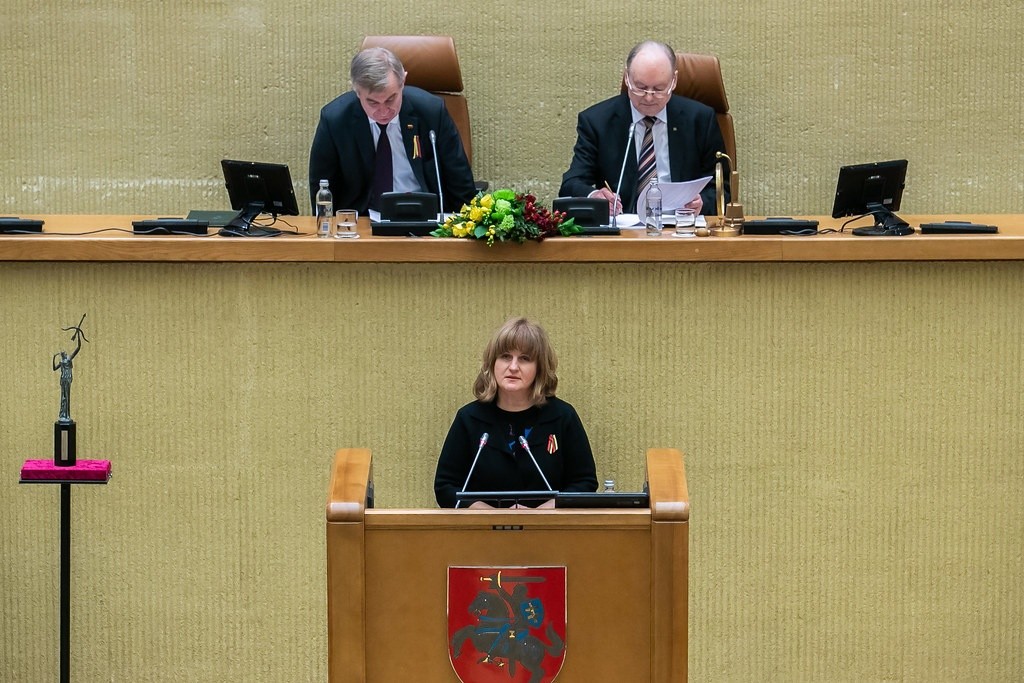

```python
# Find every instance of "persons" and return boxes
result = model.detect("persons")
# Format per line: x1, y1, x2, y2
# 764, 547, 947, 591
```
434, 316, 599, 509
308, 47, 476, 215
53, 331, 81, 418
557, 39, 731, 216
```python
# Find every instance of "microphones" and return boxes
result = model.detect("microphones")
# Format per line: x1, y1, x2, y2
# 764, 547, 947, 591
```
612, 123, 636, 227
429, 129, 444, 223
518, 435, 553, 491
454, 433, 489, 509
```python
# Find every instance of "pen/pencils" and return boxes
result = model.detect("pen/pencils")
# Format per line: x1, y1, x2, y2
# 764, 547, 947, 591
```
605, 180, 623, 214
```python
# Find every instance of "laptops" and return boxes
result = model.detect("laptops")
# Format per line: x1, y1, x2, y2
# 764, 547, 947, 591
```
186, 210, 242, 227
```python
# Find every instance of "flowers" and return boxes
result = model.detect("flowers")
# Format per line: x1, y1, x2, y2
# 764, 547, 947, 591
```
429, 187, 589, 248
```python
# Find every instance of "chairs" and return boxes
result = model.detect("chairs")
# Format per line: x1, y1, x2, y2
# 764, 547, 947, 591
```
620, 52, 737, 170
361, 35, 473, 167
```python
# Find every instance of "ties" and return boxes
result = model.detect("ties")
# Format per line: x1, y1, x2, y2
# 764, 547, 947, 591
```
636, 115, 658, 212
376, 121, 396, 193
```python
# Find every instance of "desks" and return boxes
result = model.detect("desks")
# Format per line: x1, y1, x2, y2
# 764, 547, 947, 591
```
331, 216, 783, 263
783, 213, 1024, 261
0, 208, 331, 265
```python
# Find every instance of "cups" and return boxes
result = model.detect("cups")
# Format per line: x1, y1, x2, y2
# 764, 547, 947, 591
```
675, 208, 696, 236
335, 209, 358, 239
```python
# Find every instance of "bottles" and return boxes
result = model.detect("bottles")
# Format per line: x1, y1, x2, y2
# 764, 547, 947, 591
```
645, 178, 663, 237
601, 480, 618, 494
315, 179, 334, 239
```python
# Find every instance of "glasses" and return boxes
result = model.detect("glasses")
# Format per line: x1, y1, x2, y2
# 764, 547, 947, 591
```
626, 71, 675, 98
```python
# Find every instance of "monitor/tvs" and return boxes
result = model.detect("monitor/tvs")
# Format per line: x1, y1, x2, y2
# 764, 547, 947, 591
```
832, 159, 916, 236
218, 160, 299, 237
555, 492, 649, 509
